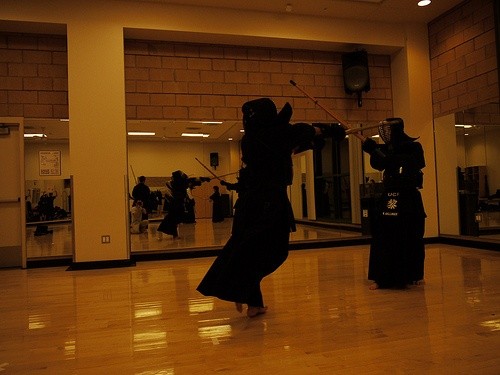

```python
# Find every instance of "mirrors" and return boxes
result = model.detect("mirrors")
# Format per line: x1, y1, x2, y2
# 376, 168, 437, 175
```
435, 103, 500, 239
126, 119, 385, 252
23, 118, 74, 256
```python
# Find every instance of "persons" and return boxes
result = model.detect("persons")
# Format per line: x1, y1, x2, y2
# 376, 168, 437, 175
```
130, 170, 211, 240
195, 98, 323, 317
24, 189, 65, 235
208, 186, 224, 223
354, 116, 429, 291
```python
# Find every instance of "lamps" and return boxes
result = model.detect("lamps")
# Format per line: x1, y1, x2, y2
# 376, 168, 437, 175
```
342, 50, 370, 108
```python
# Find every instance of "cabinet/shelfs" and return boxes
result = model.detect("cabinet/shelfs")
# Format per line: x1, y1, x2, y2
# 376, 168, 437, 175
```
192, 176, 233, 219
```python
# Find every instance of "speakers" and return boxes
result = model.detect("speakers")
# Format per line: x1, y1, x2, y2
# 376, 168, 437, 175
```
210, 152, 218, 166
341, 50, 370, 95
